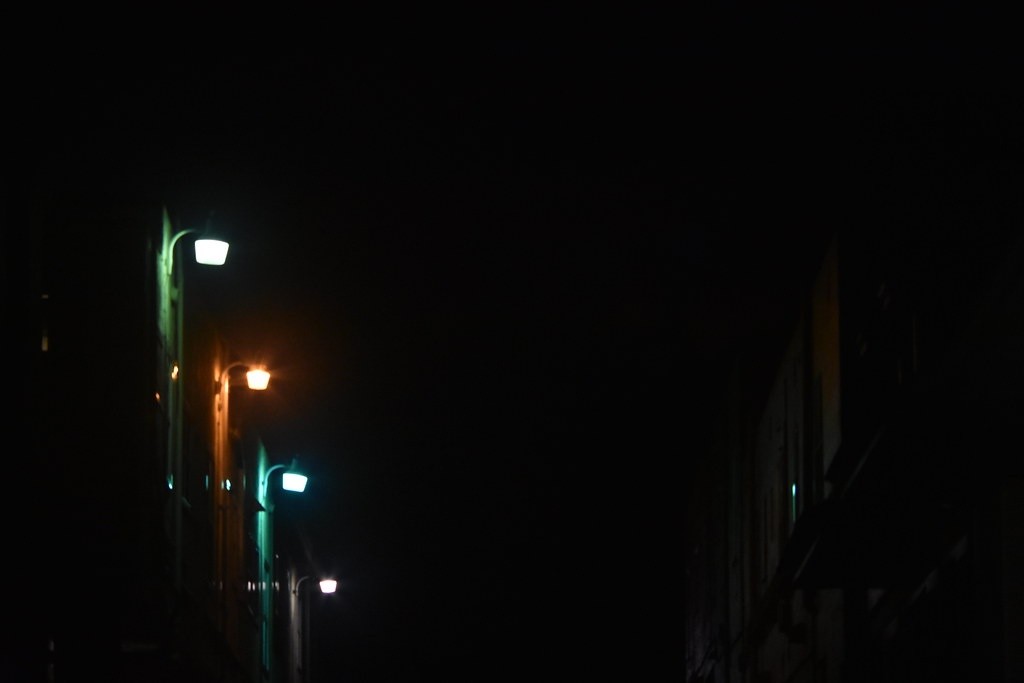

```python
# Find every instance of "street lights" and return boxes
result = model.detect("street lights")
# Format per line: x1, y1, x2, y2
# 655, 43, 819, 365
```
288, 573, 336, 682
158, 229, 229, 606
258, 463, 308, 682
212, 360, 269, 604
